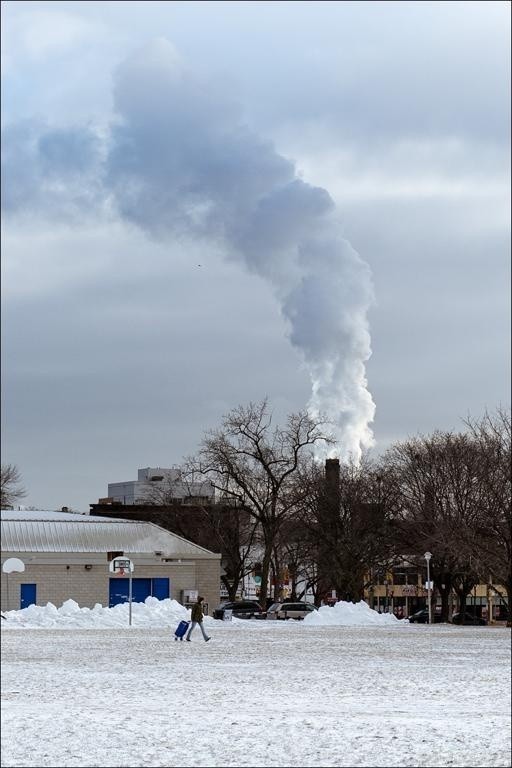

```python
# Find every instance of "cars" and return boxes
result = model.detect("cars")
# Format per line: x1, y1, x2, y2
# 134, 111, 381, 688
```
266, 602, 319, 621
448, 612, 486, 626
212, 601, 263, 621
402, 610, 443, 624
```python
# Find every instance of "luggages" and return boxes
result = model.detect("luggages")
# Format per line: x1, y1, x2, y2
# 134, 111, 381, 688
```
174, 620, 191, 640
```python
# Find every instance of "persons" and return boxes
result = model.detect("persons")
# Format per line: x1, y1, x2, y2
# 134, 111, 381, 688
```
186, 596, 211, 642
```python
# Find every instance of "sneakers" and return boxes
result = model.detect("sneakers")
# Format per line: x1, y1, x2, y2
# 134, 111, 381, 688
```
206, 637, 211, 642
187, 639, 190, 641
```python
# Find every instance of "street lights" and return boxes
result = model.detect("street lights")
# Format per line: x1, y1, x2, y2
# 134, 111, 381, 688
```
424, 552, 433, 623
